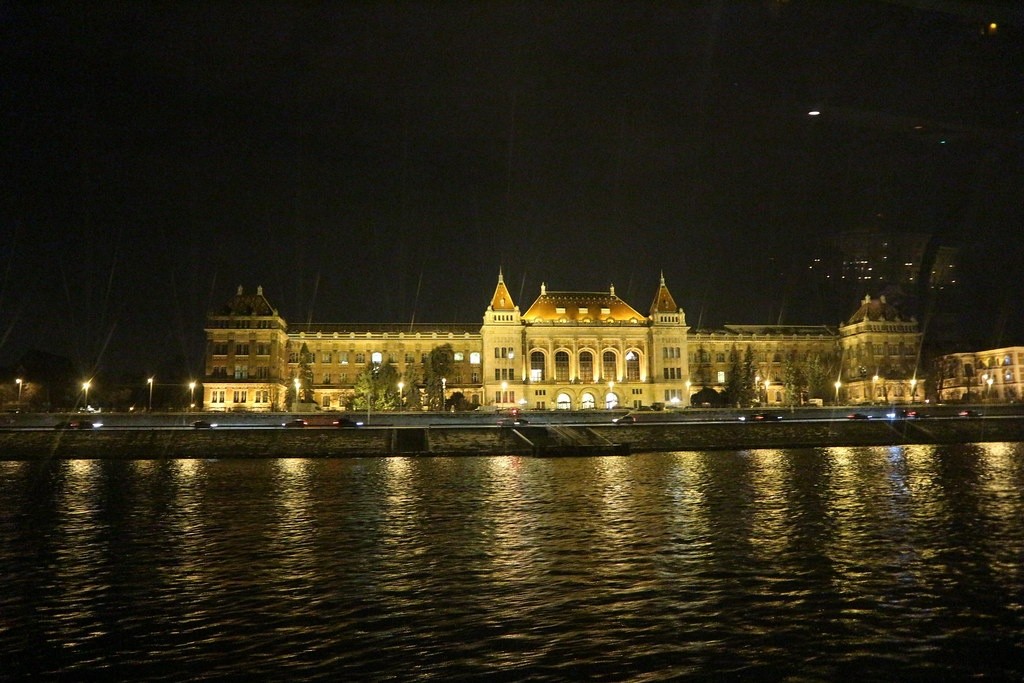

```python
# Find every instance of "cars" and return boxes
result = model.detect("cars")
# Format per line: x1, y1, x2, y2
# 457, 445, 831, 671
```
498, 418, 530, 426
189, 421, 214, 428
906, 412, 931, 418
56, 420, 94, 429
847, 414, 869, 420
738, 413, 784, 421
613, 415, 637, 424
958, 410, 981, 417
282, 419, 308, 427
332, 418, 363, 426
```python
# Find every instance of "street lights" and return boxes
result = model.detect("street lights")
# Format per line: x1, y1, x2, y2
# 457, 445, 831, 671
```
765, 379, 771, 399
911, 379, 918, 403
754, 376, 760, 393
870, 374, 879, 405
294, 377, 302, 408
83, 383, 89, 408
442, 378, 447, 411
685, 380, 691, 405
188, 382, 196, 407
397, 381, 404, 410
16, 379, 22, 402
148, 379, 153, 411
834, 382, 843, 405
